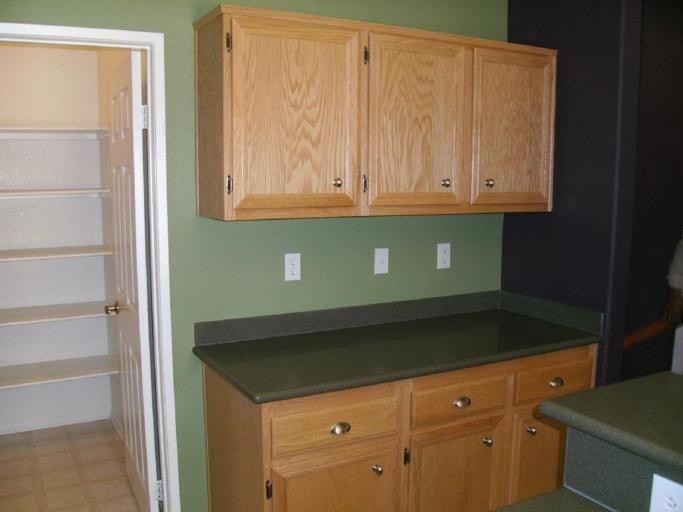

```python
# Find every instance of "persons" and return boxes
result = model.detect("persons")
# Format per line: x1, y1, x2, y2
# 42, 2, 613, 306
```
660, 238, 683, 376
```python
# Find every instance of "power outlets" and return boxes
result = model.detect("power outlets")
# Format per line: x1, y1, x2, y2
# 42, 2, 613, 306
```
435, 242, 451, 270
649, 473, 683, 512
284, 253, 300, 282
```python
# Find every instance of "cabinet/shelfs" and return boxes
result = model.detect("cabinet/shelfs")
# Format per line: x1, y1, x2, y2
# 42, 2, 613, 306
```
362, 21, 556, 218
0, 127, 119, 435
201, 361, 401, 511
191, 2, 362, 224
404, 342, 597, 510
